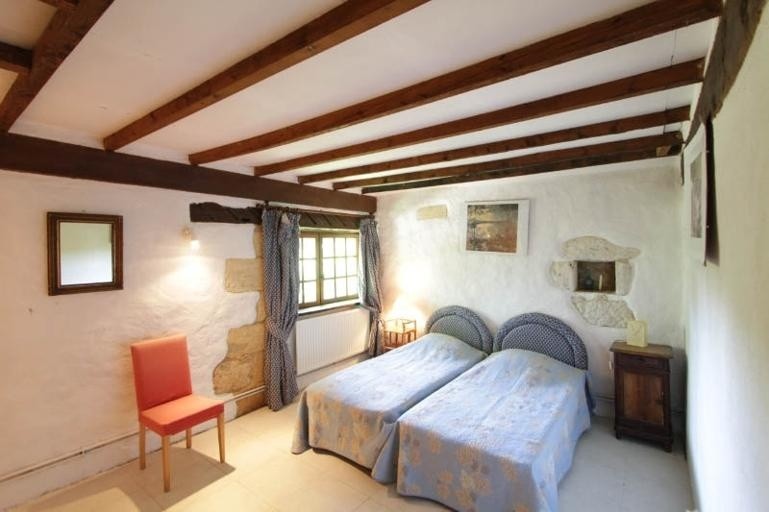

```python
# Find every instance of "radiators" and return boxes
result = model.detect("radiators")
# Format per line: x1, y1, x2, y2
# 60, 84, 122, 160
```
294, 308, 371, 377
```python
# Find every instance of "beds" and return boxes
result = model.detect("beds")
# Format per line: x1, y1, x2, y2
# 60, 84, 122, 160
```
305, 305, 492, 474
397, 313, 588, 511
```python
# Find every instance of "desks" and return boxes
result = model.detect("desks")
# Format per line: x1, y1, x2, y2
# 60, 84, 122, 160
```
609, 340, 673, 452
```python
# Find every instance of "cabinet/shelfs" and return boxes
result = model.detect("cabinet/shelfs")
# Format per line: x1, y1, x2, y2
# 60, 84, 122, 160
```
383, 318, 417, 353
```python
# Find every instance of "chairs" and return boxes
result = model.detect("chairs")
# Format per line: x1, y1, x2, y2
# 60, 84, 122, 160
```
131, 335, 226, 493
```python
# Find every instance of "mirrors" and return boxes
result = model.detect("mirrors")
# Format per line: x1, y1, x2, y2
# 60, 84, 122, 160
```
47, 212, 123, 295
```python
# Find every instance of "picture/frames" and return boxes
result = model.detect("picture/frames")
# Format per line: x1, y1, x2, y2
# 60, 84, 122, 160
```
460, 199, 530, 257
